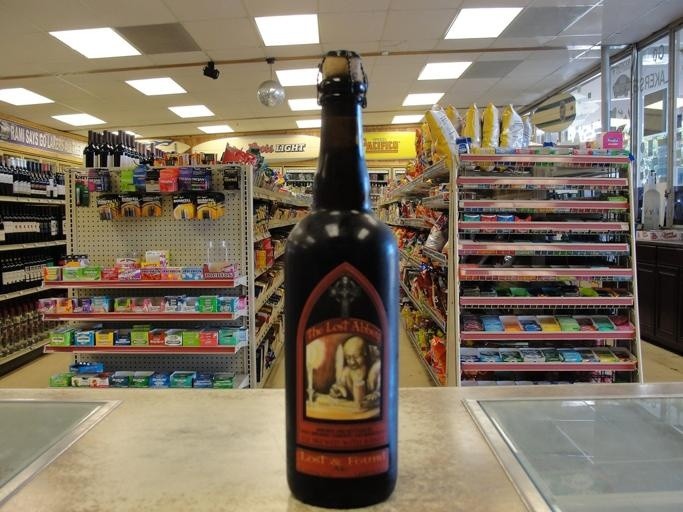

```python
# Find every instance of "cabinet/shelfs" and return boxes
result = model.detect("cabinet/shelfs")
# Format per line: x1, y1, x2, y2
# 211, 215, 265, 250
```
0, 148, 645, 387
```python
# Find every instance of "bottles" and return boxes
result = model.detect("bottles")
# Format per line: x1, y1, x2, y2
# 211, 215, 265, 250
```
0, 153, 65, 354
82, 128, 205, 173
285, 51, 401, 508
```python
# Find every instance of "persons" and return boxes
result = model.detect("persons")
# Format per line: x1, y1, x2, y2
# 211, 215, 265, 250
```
329, 336, 382, 409
